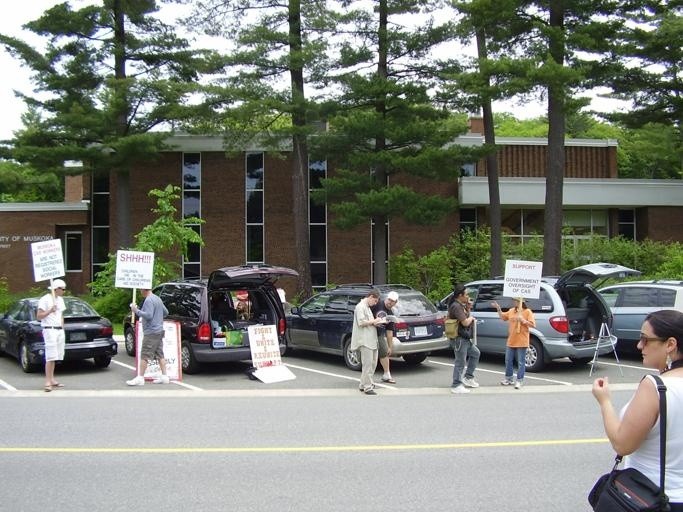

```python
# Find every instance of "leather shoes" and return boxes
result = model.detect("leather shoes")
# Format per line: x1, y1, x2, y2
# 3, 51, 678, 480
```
358, 384, 375, 391
365, 389, 377, 395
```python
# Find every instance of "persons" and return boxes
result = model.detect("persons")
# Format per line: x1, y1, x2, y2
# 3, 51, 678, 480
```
370, 292, 399, 383
446, 285, 480, 394
126, 287, 170, 385
591, 310, 683, 512
491, 296, 537, 389
38, 280, 67, 392
351, 292, 381, 395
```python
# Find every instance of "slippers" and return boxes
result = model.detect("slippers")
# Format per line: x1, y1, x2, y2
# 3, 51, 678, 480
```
381, 376, 396, 383
51, 383, 65, 388
43, 385, 52, 391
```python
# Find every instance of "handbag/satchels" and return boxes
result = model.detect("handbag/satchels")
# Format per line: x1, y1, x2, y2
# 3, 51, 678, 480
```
443, 317, 458, 339
587, 374, 671, 512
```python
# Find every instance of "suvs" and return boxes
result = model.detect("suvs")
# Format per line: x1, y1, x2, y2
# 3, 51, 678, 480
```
598, 279, 682, 354
122, 264, 299, 375
436, 263, 642, 372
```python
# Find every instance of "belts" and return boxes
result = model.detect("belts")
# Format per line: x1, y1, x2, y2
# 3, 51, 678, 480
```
42, 325, 64, 331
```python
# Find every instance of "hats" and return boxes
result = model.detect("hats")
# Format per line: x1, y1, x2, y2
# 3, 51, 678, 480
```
386, 291, 398, 302
47, 279, 66, 290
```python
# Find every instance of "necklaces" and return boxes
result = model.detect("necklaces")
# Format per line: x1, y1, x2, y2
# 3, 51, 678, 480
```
659, 358, 683, 374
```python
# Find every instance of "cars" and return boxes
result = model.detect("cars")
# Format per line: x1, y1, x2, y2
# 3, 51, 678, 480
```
285, 283, 450, 371
0, 295, 117, 373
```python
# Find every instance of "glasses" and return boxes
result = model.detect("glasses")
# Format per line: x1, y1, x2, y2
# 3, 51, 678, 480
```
638, 334, 668, 346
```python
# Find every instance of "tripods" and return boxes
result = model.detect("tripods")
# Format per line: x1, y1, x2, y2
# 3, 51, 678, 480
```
589, 323, 624, 378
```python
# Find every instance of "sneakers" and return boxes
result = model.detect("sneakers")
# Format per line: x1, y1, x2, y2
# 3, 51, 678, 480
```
153, 375, 170, 386
451, 384, 471, 395
126, 375, 145, 387
501, 379, 515, 386
514, 379, 524, 388
462, 377, 480, 388
